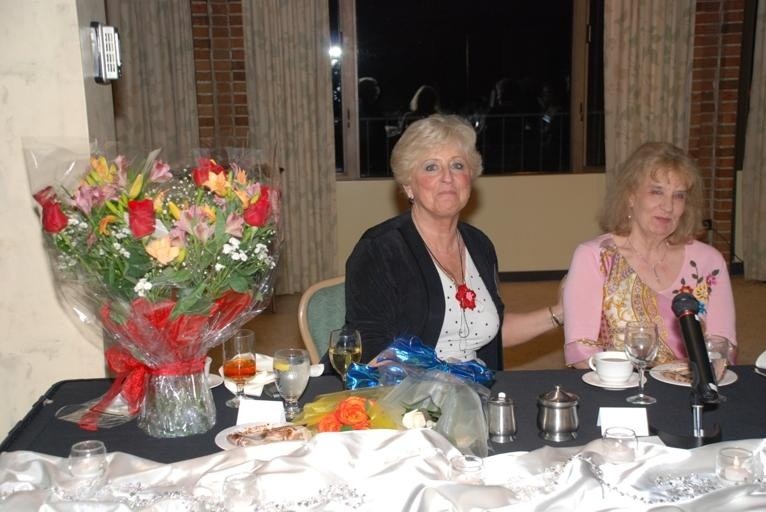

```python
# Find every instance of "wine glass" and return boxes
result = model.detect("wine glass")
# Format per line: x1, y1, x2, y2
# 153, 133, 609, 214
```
273, 348, 310, 421
222, 328, 256, 408
623, 321, 657, 406
328, 328, 362, 391
702, 334, 730, 384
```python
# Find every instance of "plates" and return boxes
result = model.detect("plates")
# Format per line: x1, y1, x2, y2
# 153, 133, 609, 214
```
647, 361, 738, 389
580, 371, 647, 391
203, 374, 222, 389
210, 421, 310, 453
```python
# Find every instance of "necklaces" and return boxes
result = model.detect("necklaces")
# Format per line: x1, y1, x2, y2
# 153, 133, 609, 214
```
625, 233, 671, 288
407, 221, 480, 341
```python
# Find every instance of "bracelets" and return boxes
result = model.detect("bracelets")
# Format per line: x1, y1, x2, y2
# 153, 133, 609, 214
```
547, 307, 565, 329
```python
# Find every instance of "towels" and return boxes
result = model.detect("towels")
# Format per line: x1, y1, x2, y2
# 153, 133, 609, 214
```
218, 352, 325, 398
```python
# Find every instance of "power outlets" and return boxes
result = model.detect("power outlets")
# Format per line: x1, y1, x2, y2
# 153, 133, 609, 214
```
703, 218, 713, 231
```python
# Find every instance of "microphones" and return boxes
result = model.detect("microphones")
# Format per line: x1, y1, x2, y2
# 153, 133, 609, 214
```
671, 293, 722, 410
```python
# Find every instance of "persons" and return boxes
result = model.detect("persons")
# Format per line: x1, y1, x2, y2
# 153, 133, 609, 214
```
404, 83, 443, 131
336, 75, 389, 173
342, 111, 569, 374
538, 77, 568, 134
485, 77, 531, 128
560, 138, 733, 384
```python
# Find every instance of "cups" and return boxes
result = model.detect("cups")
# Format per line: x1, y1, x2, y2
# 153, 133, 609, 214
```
601, 427, 639, 466
67, 440, 106, 480
588, 351, 633, 384
715, 447, 754, 488
203, 355, 211, 378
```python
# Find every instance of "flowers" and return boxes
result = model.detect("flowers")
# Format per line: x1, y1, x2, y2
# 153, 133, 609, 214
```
30, 148, 283, 436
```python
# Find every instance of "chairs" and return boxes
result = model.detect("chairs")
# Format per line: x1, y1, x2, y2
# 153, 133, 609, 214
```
295, 275, 355, 366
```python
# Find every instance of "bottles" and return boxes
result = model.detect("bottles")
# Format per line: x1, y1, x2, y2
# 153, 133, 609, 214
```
535, 384, 581, 444
484, 391, 518, 444
